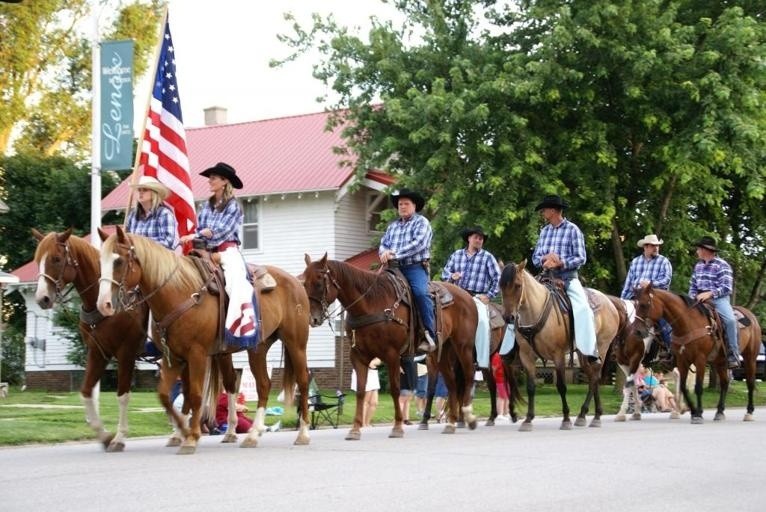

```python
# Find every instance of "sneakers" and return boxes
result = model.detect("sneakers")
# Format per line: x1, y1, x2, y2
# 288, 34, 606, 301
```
210, 426, 223, 435
418, 342, 429, 353
271, 420, 281, 433
727, 361, 738, 367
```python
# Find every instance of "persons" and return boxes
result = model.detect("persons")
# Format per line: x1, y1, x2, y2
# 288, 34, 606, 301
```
173, 382, 220, 436
624, 363, 688, 414
434, 368, 452, 422
532, 195, 603, 366
441, 225, 502, 383
394, 365, 416, 425
158, 366, 186, 423
687, 236, 741, 369
178, 161, 258, 342
121, 173, 179, 363
349, 357, 383, 430
415, 359, 435, 419
492, 351, 513, 422
214, 385, 283, 432
620, 234, 673, 352
379, 187, 437, 352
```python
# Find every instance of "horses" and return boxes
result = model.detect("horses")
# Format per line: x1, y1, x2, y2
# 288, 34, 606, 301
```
418, 299, 529, 431
29, 226, 239, 453
610, 296, 689, 422
303, 252, 479, 440
498, 258, 627, 431
631, 279, 762, 424
95, 224, 312, 456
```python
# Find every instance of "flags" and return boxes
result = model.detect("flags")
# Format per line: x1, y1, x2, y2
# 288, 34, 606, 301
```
139, 16, 201, 253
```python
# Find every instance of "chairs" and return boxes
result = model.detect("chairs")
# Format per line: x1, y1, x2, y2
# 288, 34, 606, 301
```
297, 376, 347, 430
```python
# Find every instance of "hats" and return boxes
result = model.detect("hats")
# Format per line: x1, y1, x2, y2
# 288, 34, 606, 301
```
637, 235, 664, 248
690, 236, 720, 252
129, 176, 168, 200
535, 195, 568, 211
460, 225, 488, 245
199, 163, 243, 189
390, 188, 424, 212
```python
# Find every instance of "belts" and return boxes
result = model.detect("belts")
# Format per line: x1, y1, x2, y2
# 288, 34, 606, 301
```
215, 241, 238, 252
